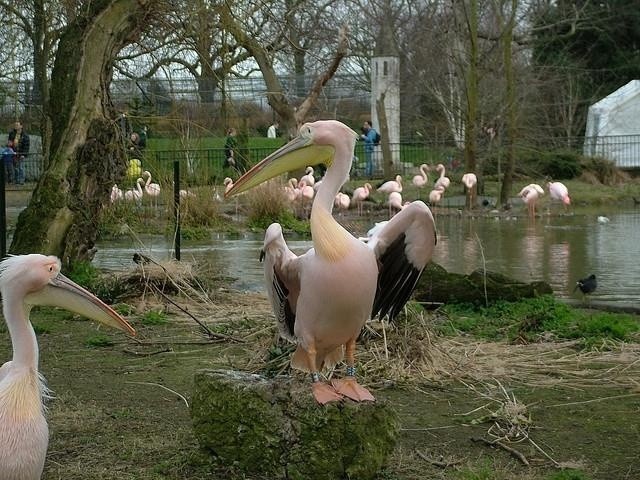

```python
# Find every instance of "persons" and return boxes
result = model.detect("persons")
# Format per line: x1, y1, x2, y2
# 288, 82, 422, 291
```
266, 120, 280, 138
359, 119, 377, 176
6, 121, 31, 185
1, 140, 25, 185
221, 127, 240, 170
128, 132, 146, 160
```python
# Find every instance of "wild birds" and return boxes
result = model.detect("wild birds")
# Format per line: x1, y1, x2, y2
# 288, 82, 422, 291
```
429, 183, 445, 211
109, 183, 125, 202
461, 172, 478, 212
547, 179, 570, 214
180, 190, 197, 200
1, 249, 136, 480
270, 164, 413, 218
220, 118, 440, 409
433, 162, 452, 198
142, 168, 161, 196
222, 174, 249, 213
211, 187, 224, 203
410, 163, 432, 200
572, 274, 598, 306
123, 176, 146, 202
519, 183, 544, 220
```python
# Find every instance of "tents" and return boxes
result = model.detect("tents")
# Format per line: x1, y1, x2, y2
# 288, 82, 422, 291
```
583, 79, 640, 169
